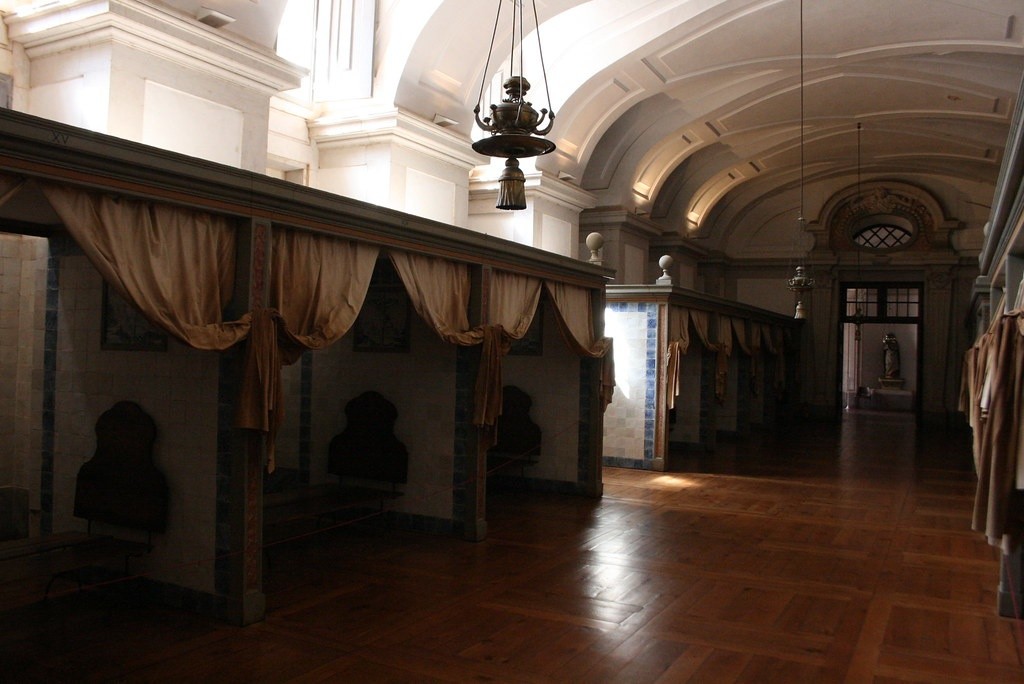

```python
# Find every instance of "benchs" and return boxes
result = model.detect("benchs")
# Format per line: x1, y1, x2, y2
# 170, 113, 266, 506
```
485, 456, 527, 479
0, 529, 152, 609
263, 481, 402, 533
871, 388, 915, 412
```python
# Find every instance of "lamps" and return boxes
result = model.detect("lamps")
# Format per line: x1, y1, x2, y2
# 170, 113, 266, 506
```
472, 0, 557, 211
788, 0, 816, 320
852, 122, 865, 324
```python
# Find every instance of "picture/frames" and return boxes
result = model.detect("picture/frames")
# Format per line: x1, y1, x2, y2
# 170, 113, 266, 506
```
506, 297, 544, 356
352, 256, 410, 353
101, 277, 168, 352
